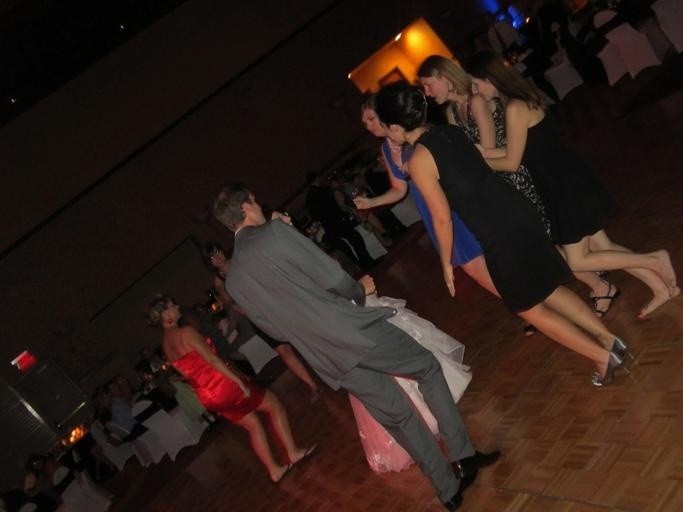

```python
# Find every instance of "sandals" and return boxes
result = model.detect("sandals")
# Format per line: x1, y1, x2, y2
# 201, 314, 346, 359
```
523, 324, 535, 337
595, 269, 609, 277
591, 280, 622, 319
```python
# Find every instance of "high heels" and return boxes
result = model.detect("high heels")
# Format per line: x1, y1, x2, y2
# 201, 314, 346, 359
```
591, 335, 635, 388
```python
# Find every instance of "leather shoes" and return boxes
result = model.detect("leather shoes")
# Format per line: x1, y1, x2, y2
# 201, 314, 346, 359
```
442, 468, 477, 512
452, 449, 502, 479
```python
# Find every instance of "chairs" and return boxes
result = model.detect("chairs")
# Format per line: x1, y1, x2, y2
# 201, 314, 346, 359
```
0, 334, 280, 511
500, 0, 682, 113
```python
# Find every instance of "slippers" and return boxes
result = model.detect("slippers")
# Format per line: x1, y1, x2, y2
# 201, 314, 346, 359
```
291, 443, 321, 467
272, 463, 292, 488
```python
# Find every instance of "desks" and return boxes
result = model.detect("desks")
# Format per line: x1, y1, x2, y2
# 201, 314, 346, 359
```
339, 191, 423, 266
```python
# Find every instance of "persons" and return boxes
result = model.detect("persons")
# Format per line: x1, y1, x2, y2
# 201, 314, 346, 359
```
143, 294, 318, 482
276, 138, 404, 275
418, 55, 619, 320
199, 241, 325, 405
375, 79, 636, 384
212, 181, 500, 511
467, 52, 680, 320
351, 95, 539, 339
469, 1, 655, 104
261, 204, 472, 474
